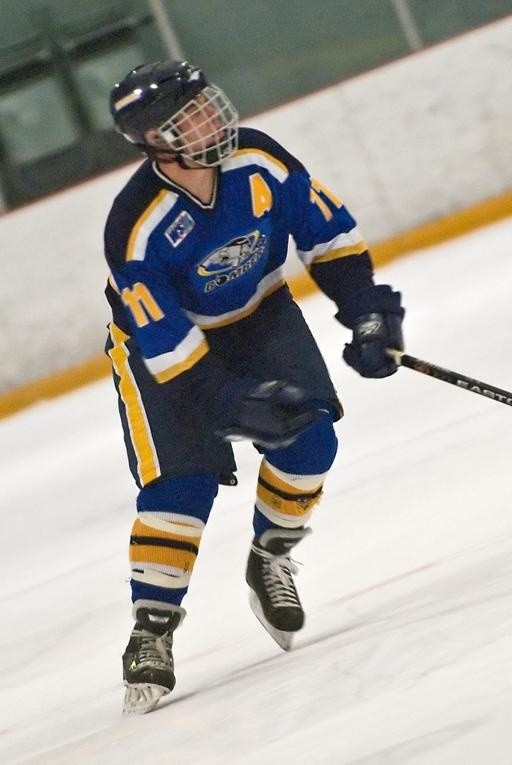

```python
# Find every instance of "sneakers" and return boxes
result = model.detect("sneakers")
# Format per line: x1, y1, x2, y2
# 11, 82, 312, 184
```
121, 598, 186, 691
245, 527, 313, 631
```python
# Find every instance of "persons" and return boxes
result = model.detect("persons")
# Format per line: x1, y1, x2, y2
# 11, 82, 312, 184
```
104, 56, 404, 695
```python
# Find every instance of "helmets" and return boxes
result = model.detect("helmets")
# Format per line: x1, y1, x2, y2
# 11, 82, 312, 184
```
109, 58, 240, 169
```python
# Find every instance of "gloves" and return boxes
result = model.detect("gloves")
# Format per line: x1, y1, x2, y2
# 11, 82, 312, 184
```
217, 379, 318, 450
335, 285, 404, 379
